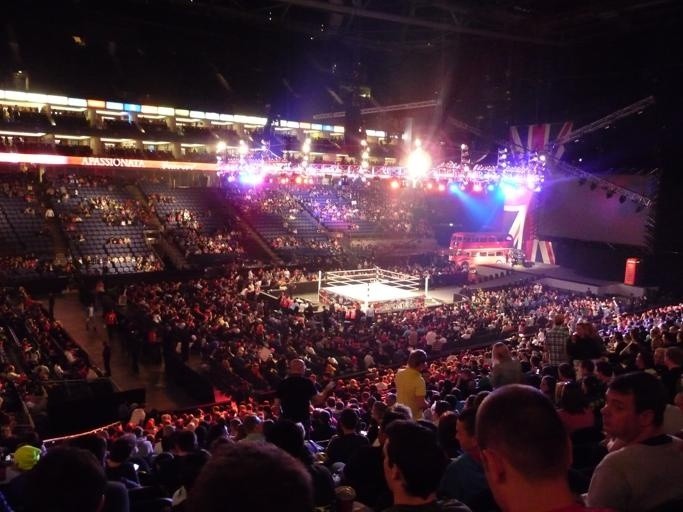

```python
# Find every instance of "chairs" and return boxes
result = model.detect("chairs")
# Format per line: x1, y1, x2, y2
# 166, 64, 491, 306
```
1, 300, 123, 512
0, 167, 438, 285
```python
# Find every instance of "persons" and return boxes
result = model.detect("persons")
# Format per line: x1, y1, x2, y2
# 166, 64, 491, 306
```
1, 101, 683, 510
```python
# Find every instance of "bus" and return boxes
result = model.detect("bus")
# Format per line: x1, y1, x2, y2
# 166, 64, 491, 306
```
447, 231, 517, 267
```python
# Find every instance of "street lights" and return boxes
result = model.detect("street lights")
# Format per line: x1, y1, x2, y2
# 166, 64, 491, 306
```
14, 67, 30, 92
